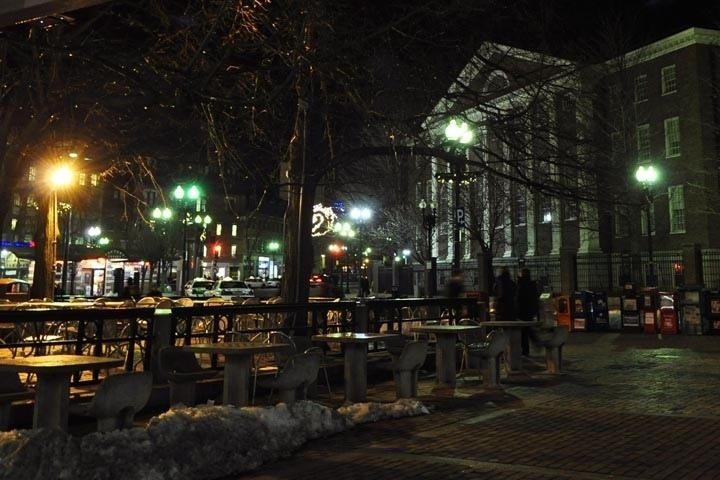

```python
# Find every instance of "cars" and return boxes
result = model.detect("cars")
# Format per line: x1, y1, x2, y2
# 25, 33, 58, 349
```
185, 276, 282, 298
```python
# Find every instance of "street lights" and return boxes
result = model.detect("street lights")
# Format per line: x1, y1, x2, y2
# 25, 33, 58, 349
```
88, 226, 110, 249
326, 206, 413, 296
635, 165, 660, 287
152, 184, 214, 297
266, 242, 283, 279
43, 163, 75, 303
418, 199, 440, 299
434, 119, 484, 298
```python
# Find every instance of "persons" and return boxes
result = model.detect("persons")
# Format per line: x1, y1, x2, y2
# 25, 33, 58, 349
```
359, 271, 370, 297
516, 268, 544, 356
492, 265, 516, 321
442, 268, 467, 298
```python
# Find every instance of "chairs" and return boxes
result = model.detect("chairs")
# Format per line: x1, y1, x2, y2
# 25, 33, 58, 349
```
0, 296, 572, 435
